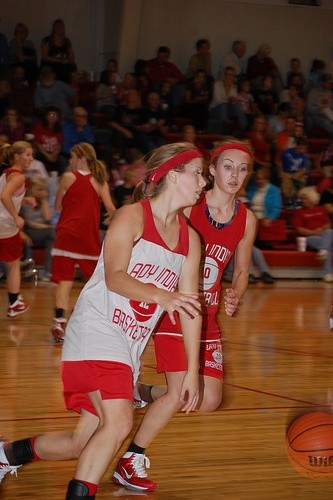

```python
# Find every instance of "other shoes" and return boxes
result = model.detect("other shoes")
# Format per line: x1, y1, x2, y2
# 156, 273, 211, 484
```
262, 272, 274, 284
51, 317, 67, 342
42, 272, 52, 281
6, 295, 29, 317
25, 268, 36, 278
324, 273, 333, 282
249, 274, 255, 284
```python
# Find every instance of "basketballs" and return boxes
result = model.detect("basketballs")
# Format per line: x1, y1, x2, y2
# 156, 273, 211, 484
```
285, 411, 333, 479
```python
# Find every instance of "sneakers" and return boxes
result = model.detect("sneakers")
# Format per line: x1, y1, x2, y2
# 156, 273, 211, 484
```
0, 438, 22, 487
111, 452, 157, 491
133, 396, 148, 409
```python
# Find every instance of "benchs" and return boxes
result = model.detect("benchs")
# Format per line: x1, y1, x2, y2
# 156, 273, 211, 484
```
163, 130, 333, 280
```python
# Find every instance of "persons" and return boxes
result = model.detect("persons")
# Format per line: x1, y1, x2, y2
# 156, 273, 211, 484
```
0, 141, 38, 316
315, 212, 333, 329
49, 142, 116, 342
0, 141, 207, 500
0, 19, 333, 253
111, 135, 259, 491
227, 246, 274, 283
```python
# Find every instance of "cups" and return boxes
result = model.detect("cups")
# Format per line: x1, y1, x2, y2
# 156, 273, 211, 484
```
296, 237, 307, 251
49, 172, 59, 196
